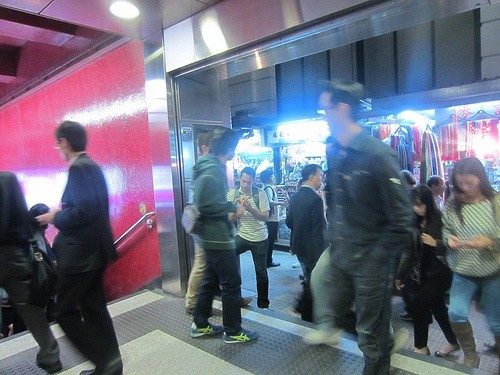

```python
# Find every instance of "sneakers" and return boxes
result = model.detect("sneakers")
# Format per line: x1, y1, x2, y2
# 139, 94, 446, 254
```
223, 328, 257, 343
189, 321, 225, 337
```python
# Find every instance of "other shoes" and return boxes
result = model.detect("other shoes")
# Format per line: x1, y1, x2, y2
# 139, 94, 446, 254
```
292, 262, 301, 268
239, 295, 253, 307
35, 353, 62, 373
434, 344, 460, 357
79, 369, 95, 375
186, 307, 198, 314
398, 311, 413, 321
415, 347, 430, 356
302, 327, 343, 347
266, 262, 281, 267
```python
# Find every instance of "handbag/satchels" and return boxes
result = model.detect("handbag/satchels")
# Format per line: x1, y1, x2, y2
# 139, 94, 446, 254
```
179, 201, 201, 234
27, 248, 59, 309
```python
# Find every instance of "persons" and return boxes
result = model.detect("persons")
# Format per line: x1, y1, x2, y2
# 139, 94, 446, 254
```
398, 170, 415, 321
284, 164, 328, 322
427, 176, 444, 214
320, 79, 410, 375
303, 246, 410, 356
406, 184, 460, 358
0, 171, 62, 373
184, 129, 258, 344
259, 168, 285, 268
35, 120, 125, 375
227, 167, 269, 312
440, 157, 500, 367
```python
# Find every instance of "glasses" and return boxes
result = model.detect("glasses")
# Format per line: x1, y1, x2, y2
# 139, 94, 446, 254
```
52, 140, 61, 150
